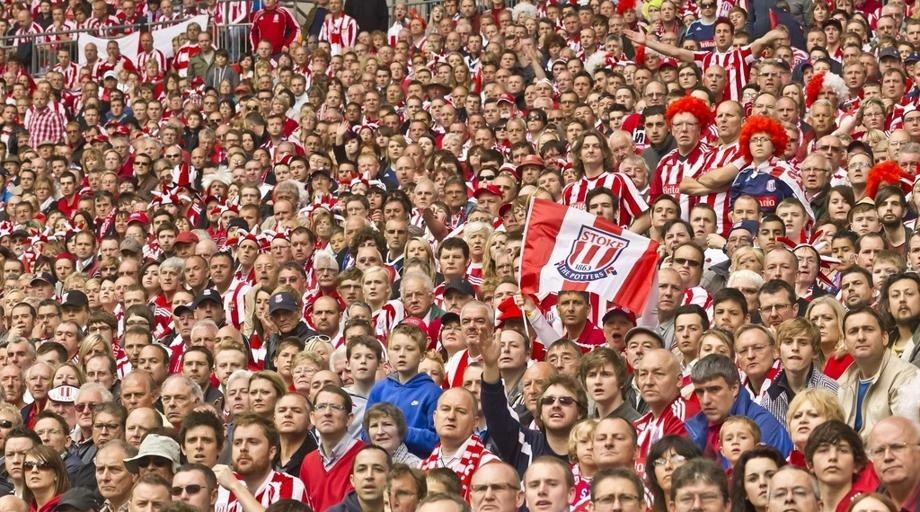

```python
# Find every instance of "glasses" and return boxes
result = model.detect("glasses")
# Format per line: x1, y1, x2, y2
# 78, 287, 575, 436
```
700, 2, 718, 10
0, 419, 11, 428
136, 456, 166, 467
756, 71, 781, 77
496, 126, 507, 131
671, 258, 704, 267
21, 460, 56, 471
478, 175, 493, 181
311, 402, 347, 413
821, 146, 839, 152
750, 137, 770, 142
527, 115, 541, 123
673, 122, 698, 128
539, 395, 584, 409
167, 484, 207, 495
652, 453, 690, 470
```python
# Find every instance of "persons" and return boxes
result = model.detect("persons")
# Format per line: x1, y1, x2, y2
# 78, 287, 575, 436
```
2, 0, 920, 511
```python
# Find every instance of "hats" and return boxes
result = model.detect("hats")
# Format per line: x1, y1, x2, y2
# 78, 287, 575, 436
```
656, 57, 679, 71
119, 239, 143, 255
821, 17, 843, 31
761, 57, 791, 70
0, 153, 20, 166
46, 381, 80, 403
56, 291, 89, 307
173, 230, 197, 247
174, 288, 223, 317
308, 166, 334, 182
602, 306, 665, 350
54, 488, 100, 507
122, 433, 182, 474
442, 279, 474, 298
497, 203, 515, 220
515, 155, 546, 175
846, 140, 873, 159
877, 46, 920, 63
726, 218, 761, 238
473, 186, 505, 199
269, 292, 300, 315
28, 274, 55, 288
90, 121, 129, 145
494, 92, 517, 106
